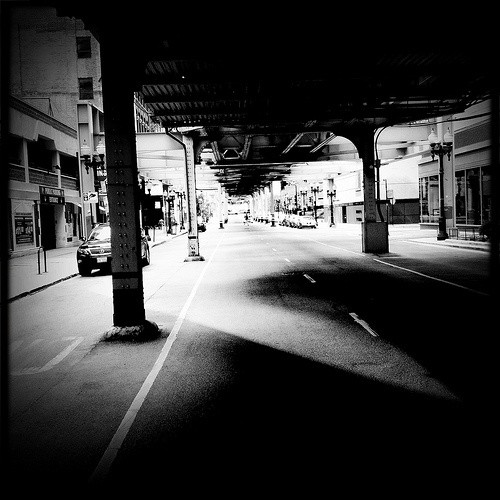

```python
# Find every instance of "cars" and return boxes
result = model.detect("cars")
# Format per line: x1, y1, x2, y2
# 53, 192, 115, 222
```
76, 222, 150, 276
252, 211, 317, 229
188, 215, 206, 232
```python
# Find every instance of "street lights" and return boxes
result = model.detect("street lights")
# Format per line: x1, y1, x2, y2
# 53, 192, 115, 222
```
300, 188, 307, 215
310, 183, 320, 226
326, 187, 337, 227
81, 138, 107, 223
427, 127, 454, 241
